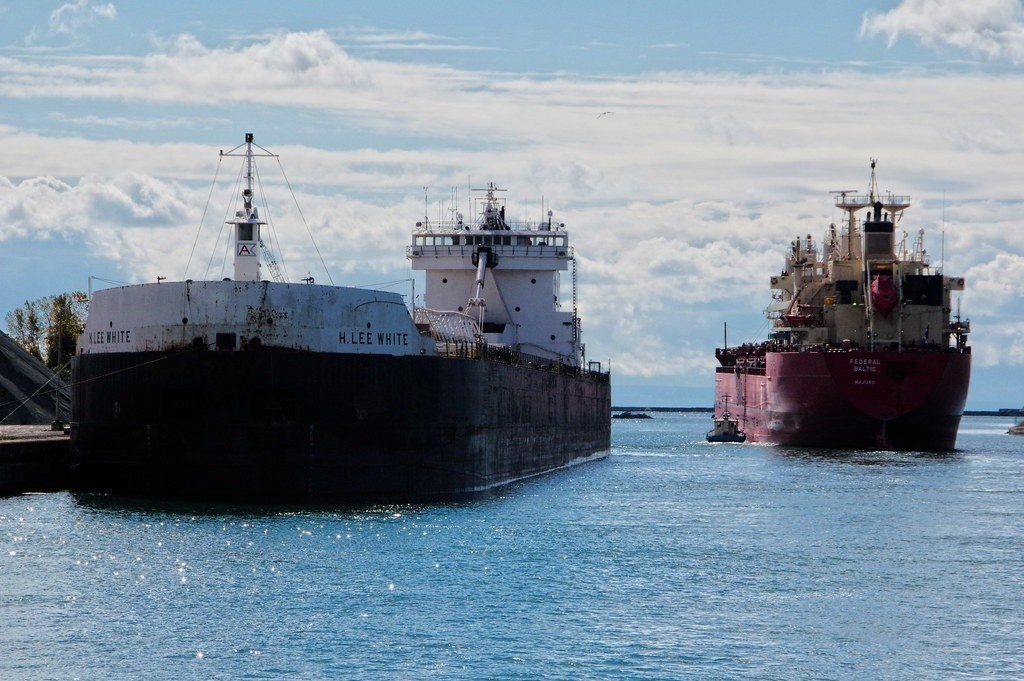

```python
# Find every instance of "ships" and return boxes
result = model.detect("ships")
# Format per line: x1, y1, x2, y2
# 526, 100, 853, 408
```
64, 130, 615, 513
704, 156, 976, 455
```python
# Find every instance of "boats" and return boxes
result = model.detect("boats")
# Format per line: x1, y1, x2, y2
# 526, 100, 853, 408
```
0, 329, 76, 501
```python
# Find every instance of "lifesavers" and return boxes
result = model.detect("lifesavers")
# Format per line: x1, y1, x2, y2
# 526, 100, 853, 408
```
957, 279, 964, 287
771, 277, 778, 285
